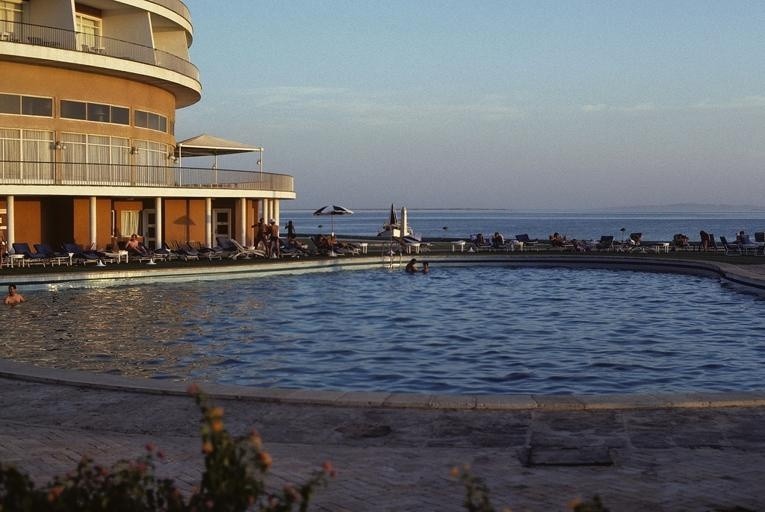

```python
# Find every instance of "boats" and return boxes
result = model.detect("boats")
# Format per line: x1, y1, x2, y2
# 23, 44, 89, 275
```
375, 199, 410, 240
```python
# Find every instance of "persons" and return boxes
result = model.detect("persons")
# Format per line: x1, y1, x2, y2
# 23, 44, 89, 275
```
422, 261, 430, 273
3, 283, 26, 305
292, 238, 313, 254
124, 233, 148, 257
492, 231, 504, 243
266, 218, 280, 260
251, 217, 268, 251
699, 230, 712, 249
320, 235, 346, 254
285, 219, 296, 242
475, 233, 488, 247
736, 231, 749, 247
676, 233, 688, 246
549, 232, 562, 242
622, 233, 643, 252
406, 258, 418, 272
339, 239, 353, 249
571, 237, 590, 253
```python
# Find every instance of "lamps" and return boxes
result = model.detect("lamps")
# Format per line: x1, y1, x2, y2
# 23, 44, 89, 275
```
54, 141, 67, 151
132, 146, 140, 155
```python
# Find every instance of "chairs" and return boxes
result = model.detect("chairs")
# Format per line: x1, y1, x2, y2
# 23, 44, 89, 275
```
119, 235, 310, 263
10, 242, 104, 269
309, 233, 353, 256
467, 230, 765, 257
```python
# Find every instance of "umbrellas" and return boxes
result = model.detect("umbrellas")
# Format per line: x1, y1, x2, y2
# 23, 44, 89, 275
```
312, 203, 354, 234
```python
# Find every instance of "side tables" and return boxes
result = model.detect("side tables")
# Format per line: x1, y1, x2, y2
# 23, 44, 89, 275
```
406, 242, 420, 254
450, 240, 465, 253
100, 250, 130, 265
351, 242, 368, 255
